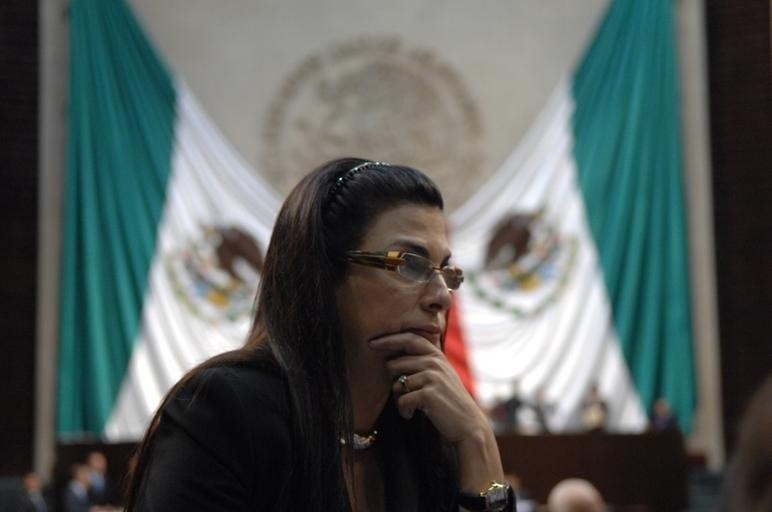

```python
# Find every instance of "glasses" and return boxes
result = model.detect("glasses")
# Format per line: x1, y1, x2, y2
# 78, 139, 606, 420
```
335, 249, 464, 290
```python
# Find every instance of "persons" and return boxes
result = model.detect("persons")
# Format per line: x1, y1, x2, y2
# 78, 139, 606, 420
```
126, 156, 522, 512
647, 395, 677, 433
574, 382, 608, 434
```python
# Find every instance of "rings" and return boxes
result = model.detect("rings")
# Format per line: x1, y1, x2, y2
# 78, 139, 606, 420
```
398, 374, 410, 392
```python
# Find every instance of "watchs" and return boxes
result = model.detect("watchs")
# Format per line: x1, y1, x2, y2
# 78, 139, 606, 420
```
457, 479, 514, 511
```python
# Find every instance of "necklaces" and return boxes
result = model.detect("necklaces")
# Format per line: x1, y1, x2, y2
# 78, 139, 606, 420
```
340, 428, 377, 450
